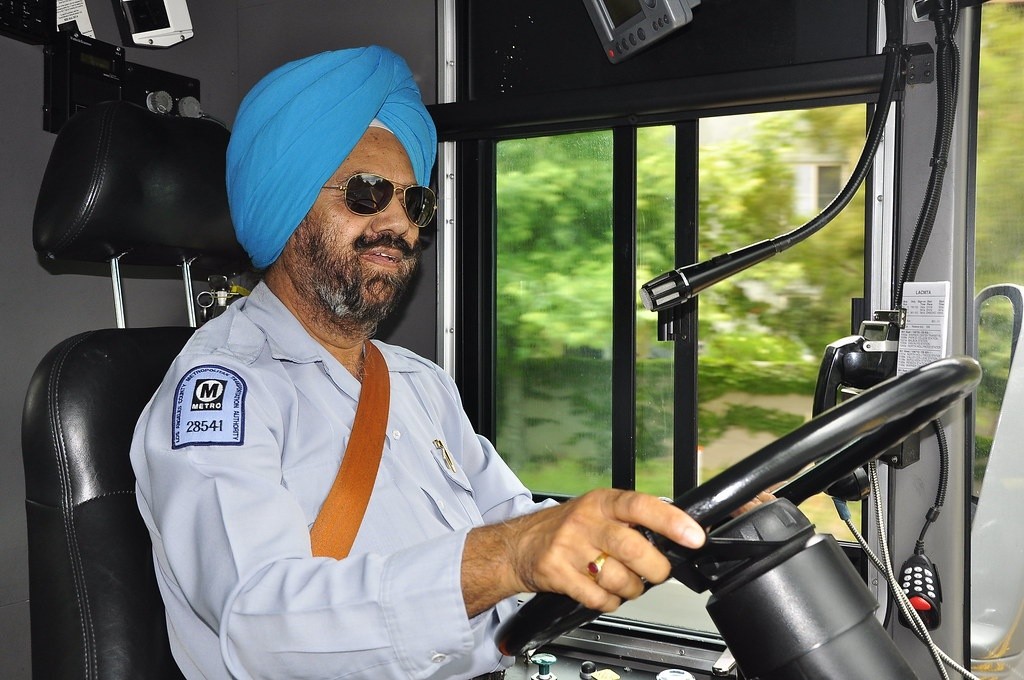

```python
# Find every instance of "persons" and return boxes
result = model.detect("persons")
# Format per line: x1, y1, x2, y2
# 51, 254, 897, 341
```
130, 45, 789, 680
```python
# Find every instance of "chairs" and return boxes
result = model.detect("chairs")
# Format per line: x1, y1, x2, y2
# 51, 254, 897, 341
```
20, 100, 240, 680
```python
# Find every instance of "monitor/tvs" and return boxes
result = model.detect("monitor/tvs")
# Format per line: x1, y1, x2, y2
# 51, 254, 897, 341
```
582, 0, 701, 64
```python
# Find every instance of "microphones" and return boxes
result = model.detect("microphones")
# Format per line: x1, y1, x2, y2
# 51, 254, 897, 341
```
639, 236, 778, 312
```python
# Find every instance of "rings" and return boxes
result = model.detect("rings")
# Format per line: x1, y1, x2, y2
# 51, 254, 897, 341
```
587, 553, 608, 583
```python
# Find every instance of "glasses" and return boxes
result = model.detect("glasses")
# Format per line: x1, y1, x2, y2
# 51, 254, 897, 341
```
321, 172, 438, 228
